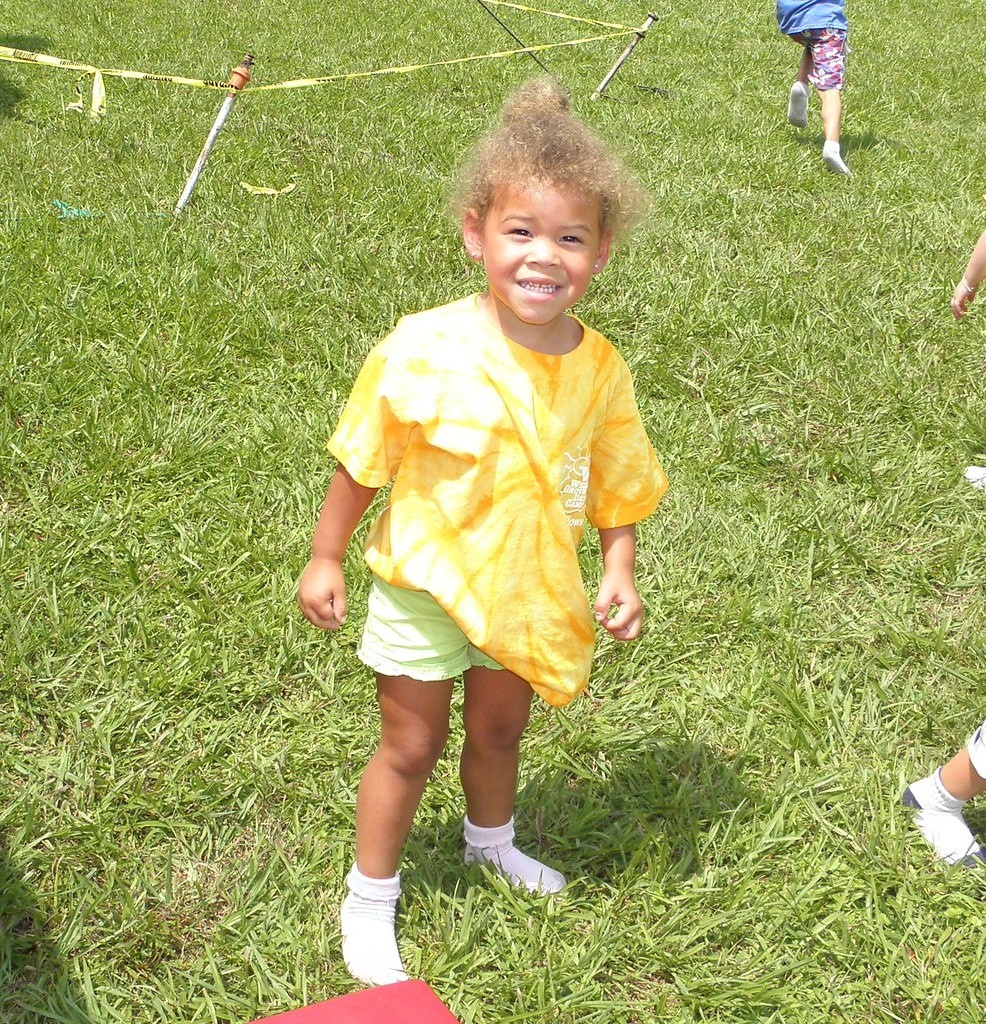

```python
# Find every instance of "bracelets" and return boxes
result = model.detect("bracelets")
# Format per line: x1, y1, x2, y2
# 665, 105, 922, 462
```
962, 275, 973, 293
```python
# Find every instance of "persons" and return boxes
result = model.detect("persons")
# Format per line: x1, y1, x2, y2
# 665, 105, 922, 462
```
902, 719, 986, 869
296, 82, 669, 986
777, 0, 851, 175
952, 228, 986, 492
